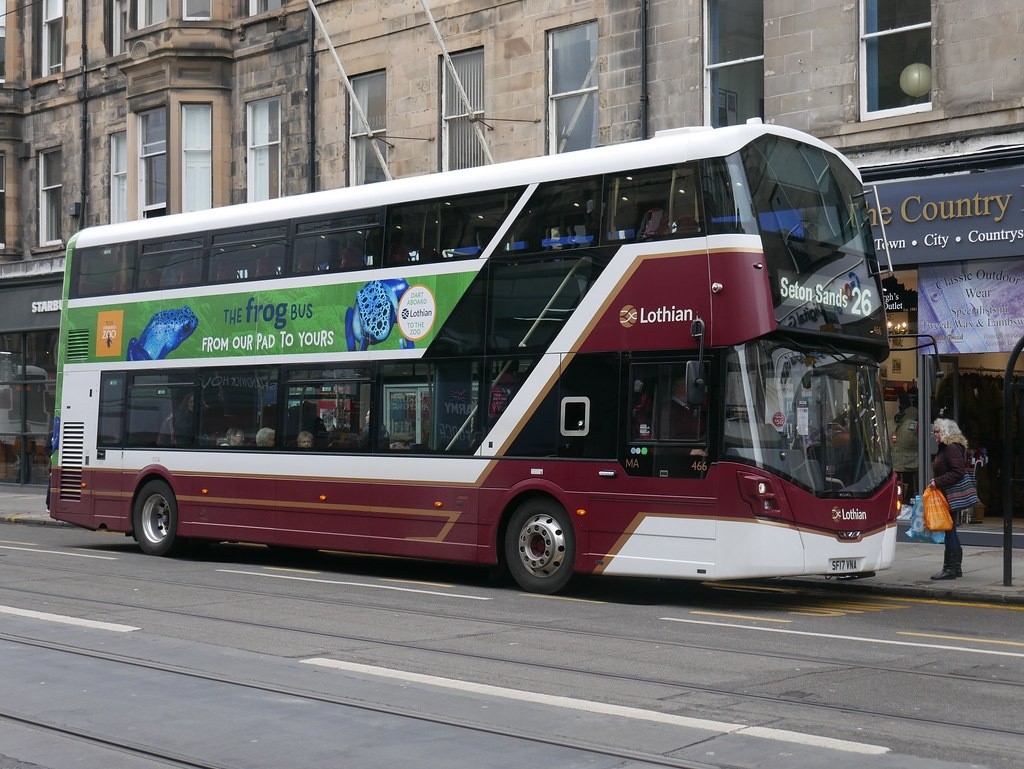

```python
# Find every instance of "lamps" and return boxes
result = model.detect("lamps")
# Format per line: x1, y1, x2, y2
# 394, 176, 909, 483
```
899, 63, 931, 103
887, 322, 908, 335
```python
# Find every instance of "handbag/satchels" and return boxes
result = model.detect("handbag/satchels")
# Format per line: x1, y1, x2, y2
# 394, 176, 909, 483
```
923, 482, 953, 530
941, 442, 981, 513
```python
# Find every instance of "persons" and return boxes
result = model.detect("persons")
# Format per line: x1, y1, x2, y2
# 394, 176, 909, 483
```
157, 392, 425, 450
15, 421, 36, 484
930, 418, 968, 580
655, 374, 711, 475
44, 430, 52, 512
886, 412, 919, 473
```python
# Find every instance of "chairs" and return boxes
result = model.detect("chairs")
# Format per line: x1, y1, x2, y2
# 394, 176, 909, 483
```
112, 243, 439, 292
639, 209, 697, 238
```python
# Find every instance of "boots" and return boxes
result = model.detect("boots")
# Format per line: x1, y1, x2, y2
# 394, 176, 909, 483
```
954, 548, 962, 576
930, 549, 956, 580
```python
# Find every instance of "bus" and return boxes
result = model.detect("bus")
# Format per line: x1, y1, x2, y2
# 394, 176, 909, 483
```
43, 118, 943, 596
0, 364, 55, 460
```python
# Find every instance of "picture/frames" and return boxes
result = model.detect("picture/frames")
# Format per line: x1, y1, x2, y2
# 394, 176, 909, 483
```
892, 358, 902, 373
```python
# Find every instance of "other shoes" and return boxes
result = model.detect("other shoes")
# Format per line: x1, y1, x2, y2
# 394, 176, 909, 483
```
905, 496, 945, 544
